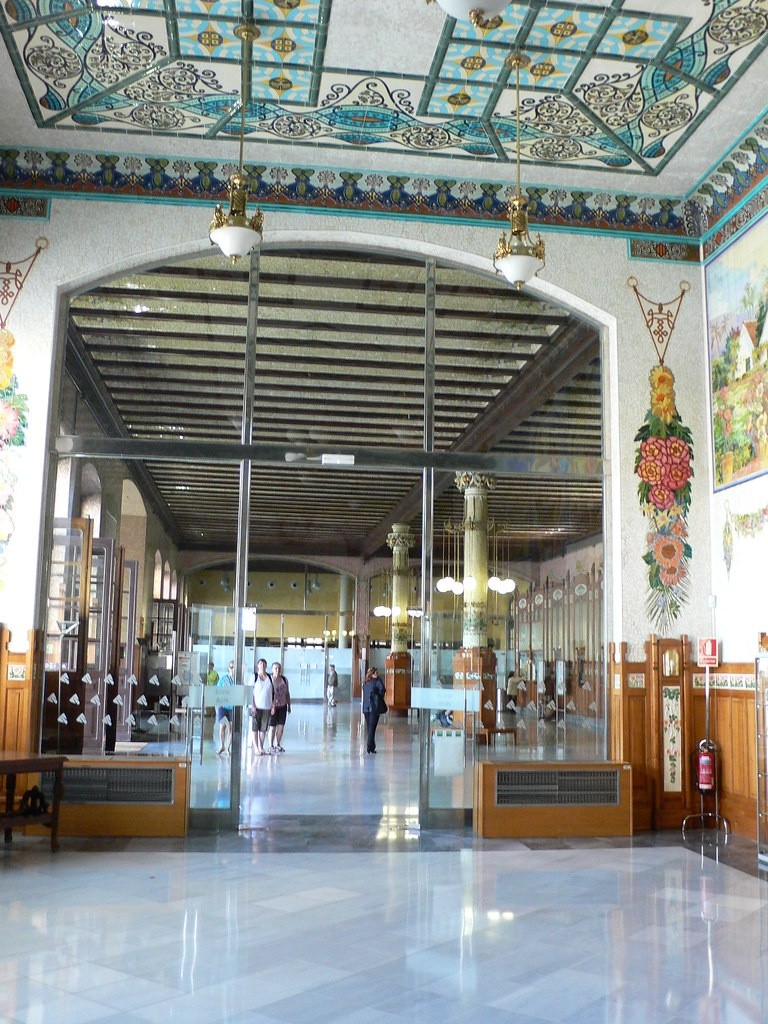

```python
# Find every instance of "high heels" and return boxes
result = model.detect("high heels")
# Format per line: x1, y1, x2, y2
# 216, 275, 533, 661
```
367, 749, 377, 754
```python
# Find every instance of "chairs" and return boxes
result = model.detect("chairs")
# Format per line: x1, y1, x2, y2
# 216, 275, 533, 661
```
0, 750, 69, 852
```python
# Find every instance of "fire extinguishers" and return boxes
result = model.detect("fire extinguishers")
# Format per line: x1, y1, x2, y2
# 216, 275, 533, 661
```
697, 744, 715, 792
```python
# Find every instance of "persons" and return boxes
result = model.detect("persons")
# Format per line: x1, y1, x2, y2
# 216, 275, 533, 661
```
362, 667, 385, 754
507, 672, 523, 714
327, 664, 338, 707
204, 659, 292, 757
545, 672, 553, 700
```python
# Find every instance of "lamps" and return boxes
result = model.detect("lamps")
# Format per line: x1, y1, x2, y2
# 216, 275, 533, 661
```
210, 25, 262, 261
437, 518, 516, 595
492, 52, 545, 290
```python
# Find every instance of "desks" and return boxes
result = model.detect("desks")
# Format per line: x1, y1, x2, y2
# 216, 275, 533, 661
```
466, 728, 518, 754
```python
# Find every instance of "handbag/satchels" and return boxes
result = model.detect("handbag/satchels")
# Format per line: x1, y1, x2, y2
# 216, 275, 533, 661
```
369, 678, 388, 715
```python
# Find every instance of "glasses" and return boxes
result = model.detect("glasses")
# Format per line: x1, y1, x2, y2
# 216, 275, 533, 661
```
229, 666, 234, 670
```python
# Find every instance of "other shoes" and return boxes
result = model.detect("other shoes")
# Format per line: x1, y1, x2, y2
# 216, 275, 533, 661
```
268, 746, 276, 752
254, 750, 263, 755
204, 713, 211, 717
260, 749, 271, 755
210, 715, 215, 720
216, 746, 225, 754
275, 745, 285, 752
226, 749, 230, 756
